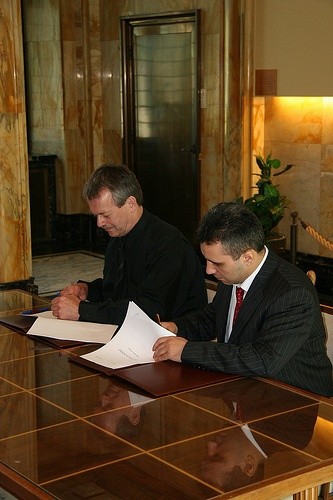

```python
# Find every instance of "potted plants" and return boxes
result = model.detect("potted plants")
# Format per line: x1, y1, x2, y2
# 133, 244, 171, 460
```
236, 153, 293, 255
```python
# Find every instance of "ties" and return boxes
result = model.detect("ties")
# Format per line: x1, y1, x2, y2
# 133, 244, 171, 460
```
234, 286, 245, 324
235, 400, 244, 421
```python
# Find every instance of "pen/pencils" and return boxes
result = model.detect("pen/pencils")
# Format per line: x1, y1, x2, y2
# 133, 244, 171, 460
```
156, 313, 160, 325
23, 307, 49, 314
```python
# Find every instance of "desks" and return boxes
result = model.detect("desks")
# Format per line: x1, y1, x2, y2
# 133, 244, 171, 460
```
0, 288, 333, 500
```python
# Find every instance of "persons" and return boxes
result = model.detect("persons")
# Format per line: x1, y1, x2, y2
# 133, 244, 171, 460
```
152, 202, 333, 399
186, 377, 319, 495
51, 162, 207, 326
92, 382, 146, 440
